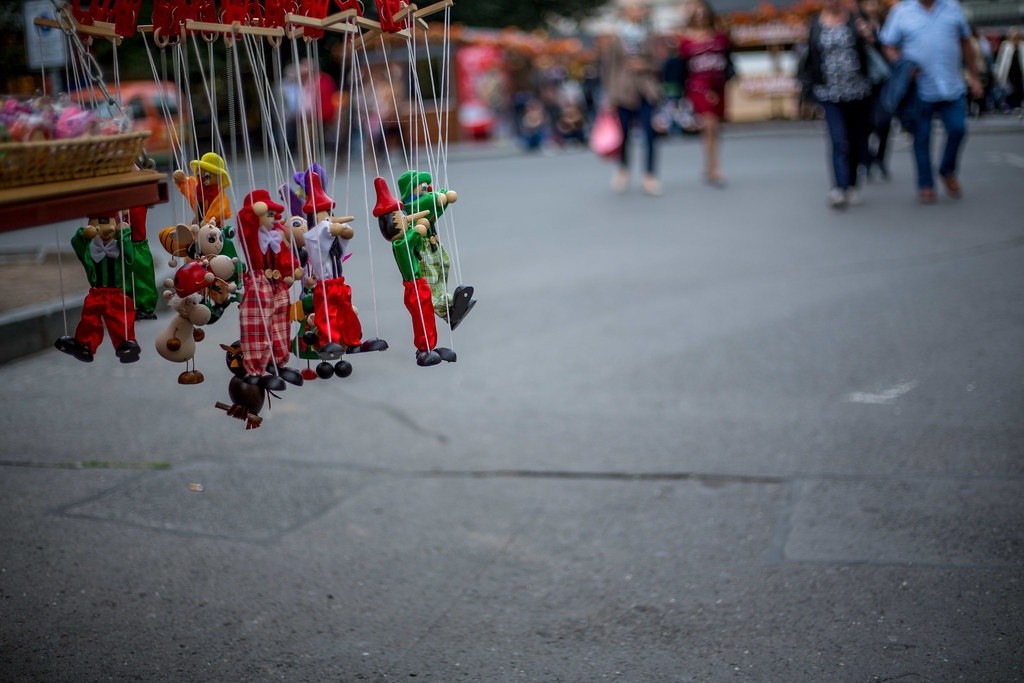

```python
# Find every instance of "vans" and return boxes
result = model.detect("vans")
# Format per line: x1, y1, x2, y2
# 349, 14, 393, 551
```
58, 79, 189, 169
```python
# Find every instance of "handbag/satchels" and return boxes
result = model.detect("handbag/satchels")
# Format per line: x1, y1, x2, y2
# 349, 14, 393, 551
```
590, 96, 622, 154
878, 59, 918, 116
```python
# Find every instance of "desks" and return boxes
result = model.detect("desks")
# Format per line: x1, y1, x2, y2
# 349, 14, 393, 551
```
0, 181, 158, 236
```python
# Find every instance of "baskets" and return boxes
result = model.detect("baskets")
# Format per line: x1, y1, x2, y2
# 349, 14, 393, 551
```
0, 130, 152, 191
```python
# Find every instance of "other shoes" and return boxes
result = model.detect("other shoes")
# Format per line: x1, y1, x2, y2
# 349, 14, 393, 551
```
641, 173, 661, 197
828, 188, 847, 207
610, 168, 629, 194
705, 167, 724, 186
943, 172, 961, 198
920, 189, 936, 204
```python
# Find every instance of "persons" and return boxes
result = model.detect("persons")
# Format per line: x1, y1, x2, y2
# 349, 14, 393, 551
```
54, 151, 477, 391
271, 0, 1024, 209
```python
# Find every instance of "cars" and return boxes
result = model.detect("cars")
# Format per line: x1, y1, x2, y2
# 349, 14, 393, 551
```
725, 49, 804, 124
